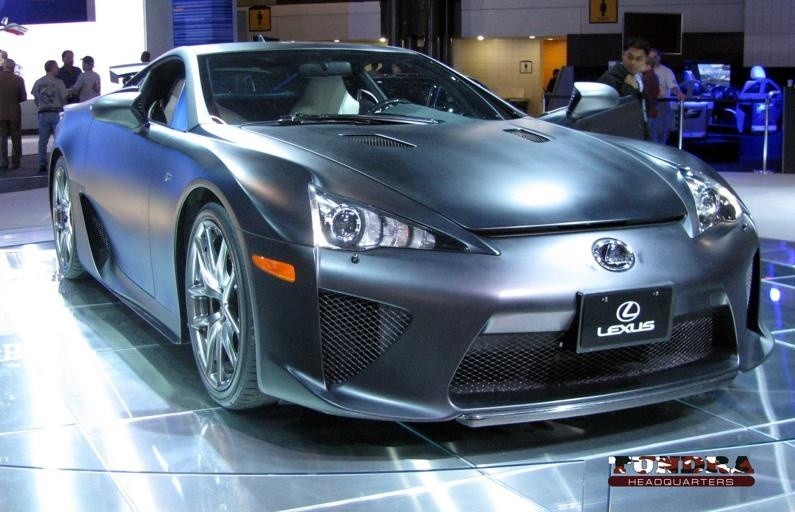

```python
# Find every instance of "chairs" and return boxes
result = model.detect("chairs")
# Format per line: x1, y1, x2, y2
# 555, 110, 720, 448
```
167, 81, 246, 134
291, 71, 359, 119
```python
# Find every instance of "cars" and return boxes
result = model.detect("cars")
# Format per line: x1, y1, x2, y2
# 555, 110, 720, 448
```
47, 35, 776, 428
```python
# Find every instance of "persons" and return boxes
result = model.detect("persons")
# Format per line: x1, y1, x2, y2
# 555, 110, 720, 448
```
140, 51, 151, 62
0, 51, 7, 67
597, 38, 651, 141
543, 68, 559, 109
69, 56, 101, 105
648, 47, 688, 145
30, 60, 69, 173
0, 57, 26, 172
54, 51, 82, 104
642, 56, 659, 144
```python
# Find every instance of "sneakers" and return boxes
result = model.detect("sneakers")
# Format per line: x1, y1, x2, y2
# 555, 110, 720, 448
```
39, 165, 48, 172
0, 161, 21, 170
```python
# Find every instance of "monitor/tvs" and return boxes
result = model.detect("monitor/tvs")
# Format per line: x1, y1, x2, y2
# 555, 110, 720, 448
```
622, 10, 683, 55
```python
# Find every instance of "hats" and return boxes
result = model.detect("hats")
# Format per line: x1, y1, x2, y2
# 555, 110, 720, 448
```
79, 55, 95, 65
